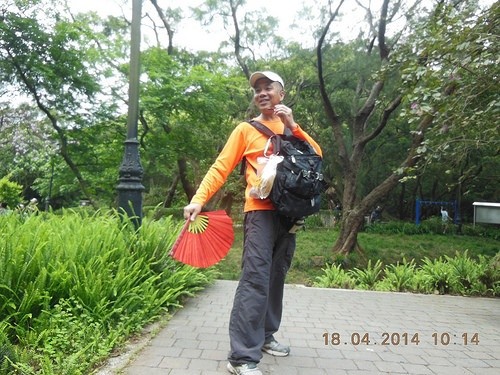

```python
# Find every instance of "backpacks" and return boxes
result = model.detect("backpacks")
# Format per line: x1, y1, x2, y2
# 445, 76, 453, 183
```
241, 119, 327, 219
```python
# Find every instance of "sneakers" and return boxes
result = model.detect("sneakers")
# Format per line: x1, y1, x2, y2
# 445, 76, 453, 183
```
262, 339, 290, 355
227, 362, 262, 375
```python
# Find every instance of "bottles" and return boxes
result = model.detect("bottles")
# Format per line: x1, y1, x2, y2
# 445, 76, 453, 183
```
255, 155, 277, 198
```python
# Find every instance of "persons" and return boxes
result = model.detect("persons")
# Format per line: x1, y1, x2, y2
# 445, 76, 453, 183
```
440, 206, 452, 222
183, 70, 323, 375
371, 206, 382, 222
26, 198, 37, 211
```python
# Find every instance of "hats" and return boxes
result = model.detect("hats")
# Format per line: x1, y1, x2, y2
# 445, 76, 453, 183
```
250, 72, 284, 91
